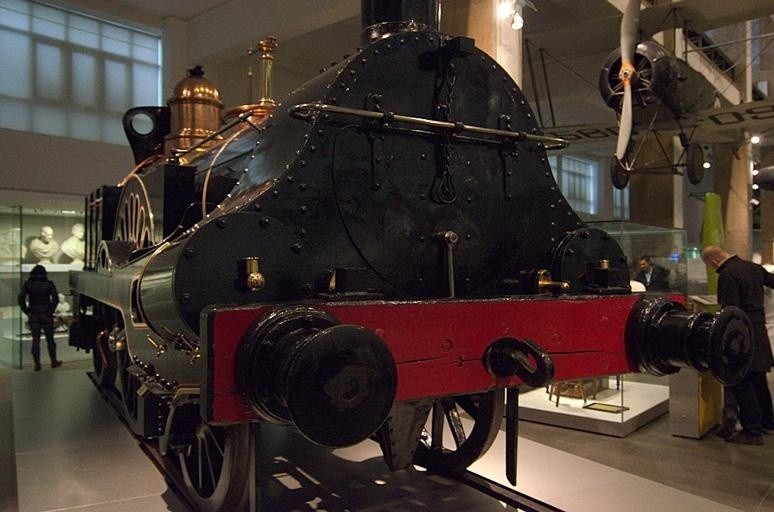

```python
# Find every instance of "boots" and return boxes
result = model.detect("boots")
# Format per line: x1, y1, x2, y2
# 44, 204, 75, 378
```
31, 344, 42, 371
47, 343, 63, 368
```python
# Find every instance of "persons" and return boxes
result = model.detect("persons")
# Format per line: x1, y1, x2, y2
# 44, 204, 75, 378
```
18, 265, 63, 371
27, 225, 58, 264
700, 246, 774, 444
55, 292, 70, 312
0, 226, 25, 261
633, 255, 672, 291
59, 222, 86, 264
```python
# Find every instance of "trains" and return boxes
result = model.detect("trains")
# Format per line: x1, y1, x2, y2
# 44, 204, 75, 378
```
67, 3, 757, 509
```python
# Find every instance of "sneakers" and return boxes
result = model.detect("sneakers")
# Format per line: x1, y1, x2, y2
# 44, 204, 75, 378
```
716, 409, 737, 439
726, 430, 764, 445
759, 423, 774, 434
